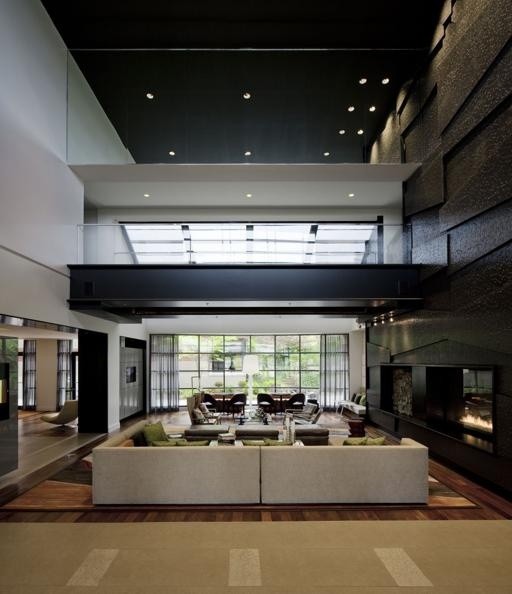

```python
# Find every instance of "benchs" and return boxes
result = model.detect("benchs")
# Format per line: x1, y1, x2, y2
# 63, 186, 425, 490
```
339, 400, 367, 419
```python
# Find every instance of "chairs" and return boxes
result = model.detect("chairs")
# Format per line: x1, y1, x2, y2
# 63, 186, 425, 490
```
187, 391, 323, 424
40, 400, 79, 433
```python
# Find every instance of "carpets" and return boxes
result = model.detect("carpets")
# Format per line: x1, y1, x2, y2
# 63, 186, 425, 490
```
2, 424, 481, 511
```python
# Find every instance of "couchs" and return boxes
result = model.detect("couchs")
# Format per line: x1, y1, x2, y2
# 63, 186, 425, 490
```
91, 420, 429, 505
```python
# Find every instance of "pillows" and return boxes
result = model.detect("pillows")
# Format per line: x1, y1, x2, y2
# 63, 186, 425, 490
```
354, 393, 361, 404
359, 394, 367, 405
350, 393, 356, 401
